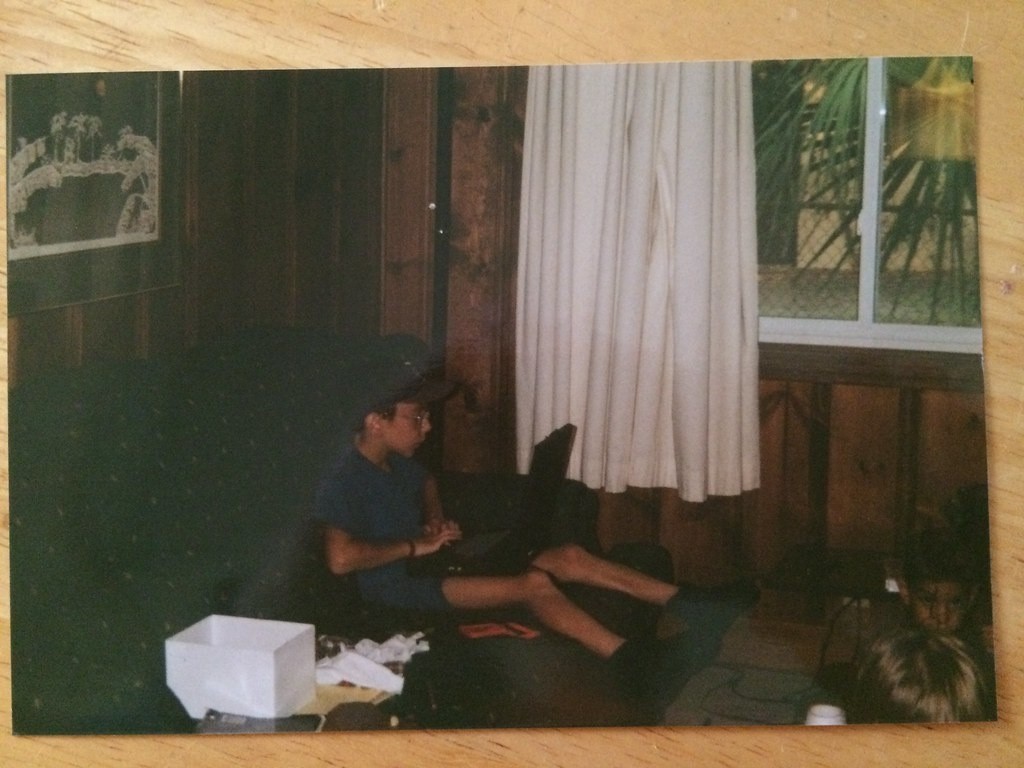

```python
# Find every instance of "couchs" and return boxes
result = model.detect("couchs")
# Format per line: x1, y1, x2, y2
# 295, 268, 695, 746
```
8, 326, 597, 731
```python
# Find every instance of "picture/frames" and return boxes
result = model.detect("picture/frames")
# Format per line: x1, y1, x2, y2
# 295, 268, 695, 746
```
6, 72, 185, 319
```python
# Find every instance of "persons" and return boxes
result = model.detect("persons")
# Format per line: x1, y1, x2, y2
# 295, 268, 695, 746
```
841, 532, 997, 724
315, 357, 741, 686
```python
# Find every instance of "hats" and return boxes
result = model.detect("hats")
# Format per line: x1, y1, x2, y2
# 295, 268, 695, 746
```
360, 334, 447, 407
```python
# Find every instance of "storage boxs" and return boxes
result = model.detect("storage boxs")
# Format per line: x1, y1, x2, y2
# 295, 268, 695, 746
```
164, 614, 316, 720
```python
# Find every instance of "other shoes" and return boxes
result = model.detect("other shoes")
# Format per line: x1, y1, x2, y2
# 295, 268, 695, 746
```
667, 582, 759, 635
630, 629, 721, 695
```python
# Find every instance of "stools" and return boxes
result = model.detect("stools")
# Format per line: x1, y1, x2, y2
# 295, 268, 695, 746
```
567, 542, 673, 657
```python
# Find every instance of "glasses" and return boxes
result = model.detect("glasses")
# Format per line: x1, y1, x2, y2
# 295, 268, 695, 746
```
384, 408, 430, 429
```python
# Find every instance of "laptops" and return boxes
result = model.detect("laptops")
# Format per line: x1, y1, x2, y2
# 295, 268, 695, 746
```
408, 424, 577, 582
